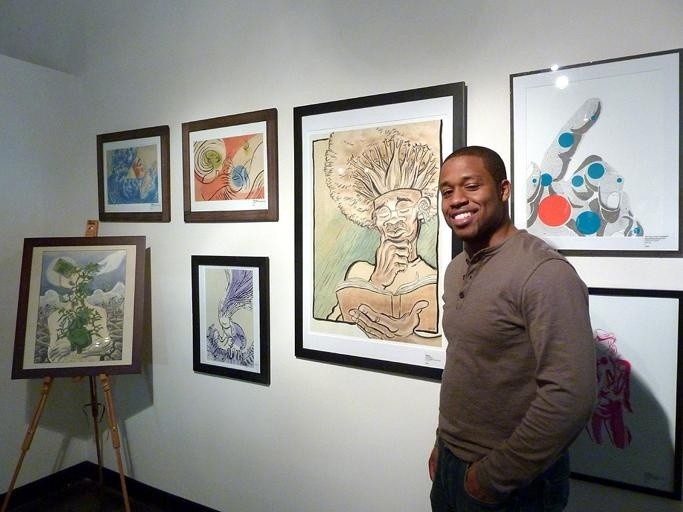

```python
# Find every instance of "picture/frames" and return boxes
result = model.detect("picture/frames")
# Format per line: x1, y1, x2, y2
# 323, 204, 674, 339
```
182, 108, 278, 223
11, 236, 146, 379
191, 255, 270, 385
568, 287, 683, 500
294, 82, 467, 382
510, 48, 683, 258
96, 125, 170, 222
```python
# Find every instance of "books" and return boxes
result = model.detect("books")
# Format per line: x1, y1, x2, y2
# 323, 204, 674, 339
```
334, 273, 439, 334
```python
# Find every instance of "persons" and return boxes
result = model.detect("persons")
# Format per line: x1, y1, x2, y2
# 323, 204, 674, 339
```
428, 145, 599, 512
323, 118, 443, 346
